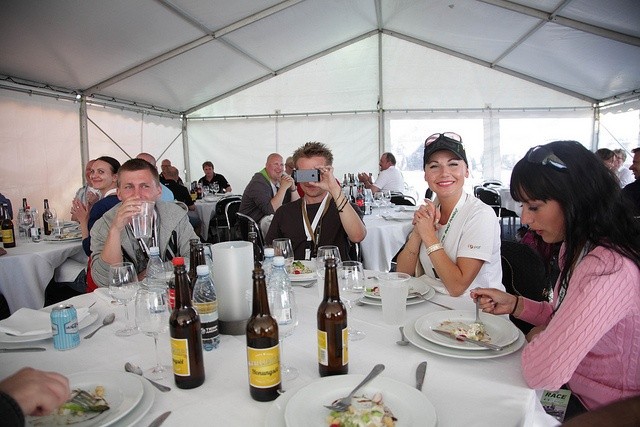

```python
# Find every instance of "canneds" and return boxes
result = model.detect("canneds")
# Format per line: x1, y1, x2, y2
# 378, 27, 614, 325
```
50, 303, 80, 350
31, 227, 42, 243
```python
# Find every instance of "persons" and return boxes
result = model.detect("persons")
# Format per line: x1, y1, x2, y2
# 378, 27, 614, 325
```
594, 148, 615, 170
357, 152, 404, 195
623, 146, 640, 225
89, 158, 212, 289
610, 148, 636, 189
136, 153, 175, 202
284, 157, 296, 176
471, 139, 640, 427
238, 153, 302, 245
71, 160, 96, 224
198, 161, 232, 193
161, 167, 196, 210
63, 156, 122, 293
0, 367, 71, 427
159, 159, 184, 186
396, 132, 510, 320
264, 142, 367, 262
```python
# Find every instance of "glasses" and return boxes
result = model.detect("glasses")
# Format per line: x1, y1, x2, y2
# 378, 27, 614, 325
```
525, 145, 573, 175
425, 131, 462, 146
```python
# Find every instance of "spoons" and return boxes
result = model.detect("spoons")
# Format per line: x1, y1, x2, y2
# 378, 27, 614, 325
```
83, 313, 115, 339
396, 327, 408, 346
125, 362, 171, 392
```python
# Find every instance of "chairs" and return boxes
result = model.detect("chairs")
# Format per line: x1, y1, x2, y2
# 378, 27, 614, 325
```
209, 195, 242, 243
474, 187, 501, 221
481, 180, 502, 187
225, 200, 242, 241
235, 212, 265, 247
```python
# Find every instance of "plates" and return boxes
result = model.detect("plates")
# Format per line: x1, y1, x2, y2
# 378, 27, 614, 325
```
284, 374, 437, 427
402, 206, 419, 211
203, 198, 219, 202
24, 370, 144, 426
359, 288, 435, 306
364, 279, 430, 299
44, 235, 82, 242
382, 212, 414, 220
53, 221, 79, 229
403, 314, 525, 360
142, 270, 189, 287
0, 309, 98, 343
415, 310, 519, 351
262, 260, 324, 281
107, 372, 155, 426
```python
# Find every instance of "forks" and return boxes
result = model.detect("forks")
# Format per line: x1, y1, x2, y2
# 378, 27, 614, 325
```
473, 287, 486, 330
292, 281, 316, 288
323, 364, 385, 412
411, 292, 455, 310
67, 388, 98, 408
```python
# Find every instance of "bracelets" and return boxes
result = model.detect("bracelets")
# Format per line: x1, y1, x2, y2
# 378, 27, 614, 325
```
338, 199, 348, 213
335, 188, 342, 202
336, 195, 346, 209
290, 186, 297, 192
426, 243, 444, 255
510, 294, 519, 315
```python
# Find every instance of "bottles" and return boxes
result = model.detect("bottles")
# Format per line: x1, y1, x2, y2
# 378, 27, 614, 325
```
188, 239, 199, 278
197, 184, 202, 200
146, 247, 166, 313
169, 264, 205, 390
42, 199, 53, 235
31, 208, 40, 242
348, 173, 357, 195
365, 190, 373, 215
342, 174, 348, 188
348, 173, 350, 185
0, 203, 3, 242
260, 248, 274, 285
356, 190, 365, 215
168, 257, 193, 311
346, 186, 355, 204
247, 269, 281, 401
317, 258, 347, 377
249, 232, 263, 269
190, 247, 206, 305
1, 203, 16, 248
193, 266, 220, 351
266, 256, 299, 337
16, 207, 26, 238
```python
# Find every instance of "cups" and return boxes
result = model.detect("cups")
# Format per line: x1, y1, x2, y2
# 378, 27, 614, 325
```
132, 201, 156, 238
377, 272, 411, 326
210, 241, 255, 336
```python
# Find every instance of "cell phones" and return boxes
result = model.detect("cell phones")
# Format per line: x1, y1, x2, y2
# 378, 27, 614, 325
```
294, 170, 320, 183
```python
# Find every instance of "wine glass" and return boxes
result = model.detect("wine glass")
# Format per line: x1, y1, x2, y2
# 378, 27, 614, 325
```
317, 246, 345, 282
373, 192, 385, 217
210, 185, 214, 195
203, 186, 209, 197
214, 184, 219, 197
336, 260, 367, 337
18, 212, 34, 244
272, 238, 294, 266
44, 208, 57, 239
135, 286, 173, 380
382, 191, 391, 214
107, 262, 141, 337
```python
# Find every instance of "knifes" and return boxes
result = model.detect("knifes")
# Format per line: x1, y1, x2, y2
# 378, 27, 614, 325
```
0, 347, 46, 353
148, 411, 171, 427
416, 361, 427, 391
432, 329, 503, 351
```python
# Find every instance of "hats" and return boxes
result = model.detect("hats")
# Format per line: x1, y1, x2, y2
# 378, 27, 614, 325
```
424, 135, 468, 166
